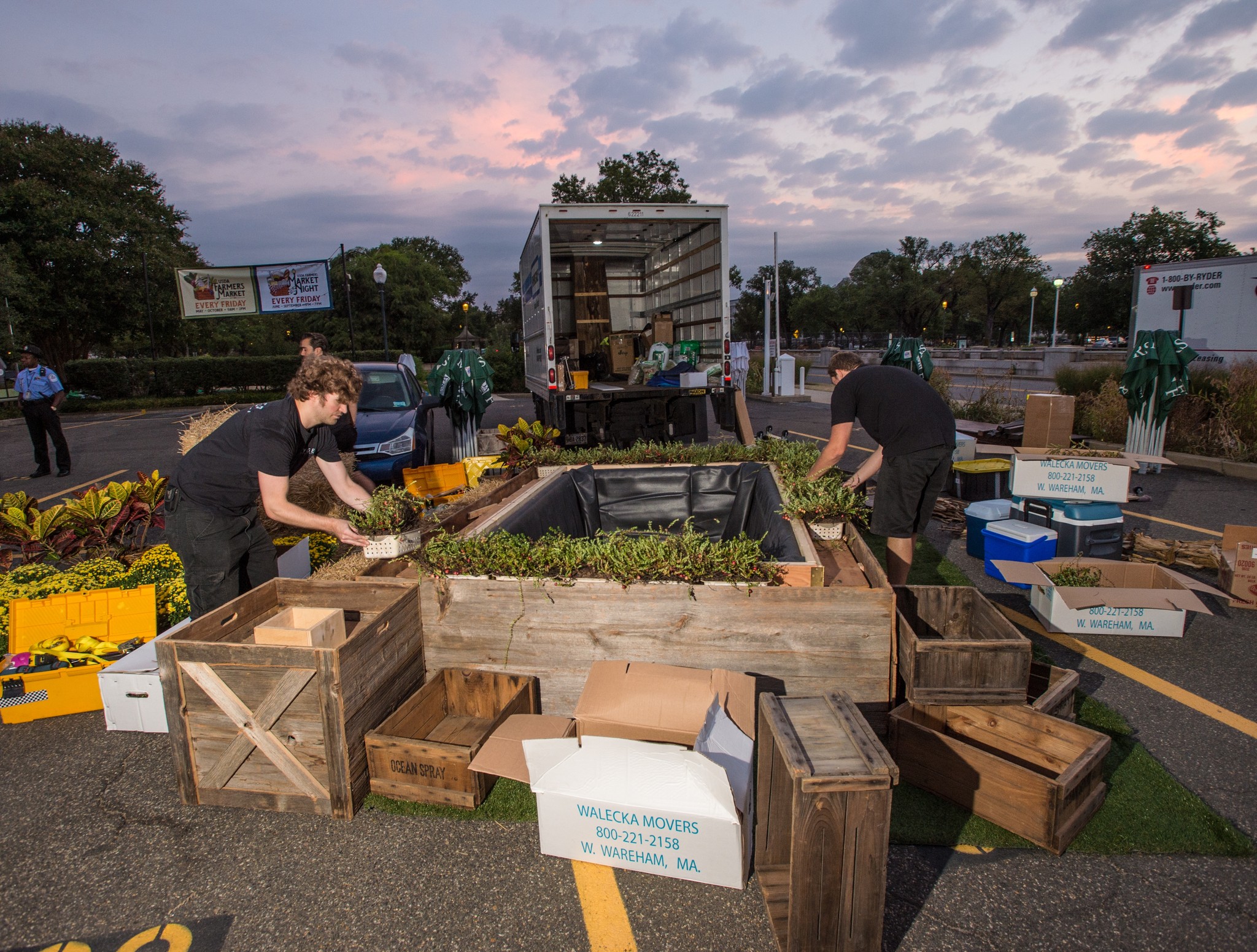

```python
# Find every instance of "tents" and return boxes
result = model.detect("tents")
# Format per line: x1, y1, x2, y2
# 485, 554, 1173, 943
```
879, 337, 934, 383
731, 342, 750, 402
1118, 329, 1199, 476
397, 353, 417, 376
426, 349, 495, 464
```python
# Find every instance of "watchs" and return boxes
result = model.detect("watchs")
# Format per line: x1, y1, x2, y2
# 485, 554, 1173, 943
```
51, 406, 57, 411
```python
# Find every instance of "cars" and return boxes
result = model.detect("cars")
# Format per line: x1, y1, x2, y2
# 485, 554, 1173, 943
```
854, 345, 865, 348
1062, 339, 1072, 343
1092, 336, 1128, 348
1040, 340, 1049, 344
337, 361, 442, 491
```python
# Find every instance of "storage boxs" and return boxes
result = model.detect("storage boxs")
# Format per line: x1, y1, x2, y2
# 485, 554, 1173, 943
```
608, 332, 633, 375
363, 666, 542, 813
734, 384, 756, 446
990, 557, 1236, 638
467, 660, 757, 889
949, 393, 1178, 591
891, 583, 1033, 706
555, 339, 589, 393
680, 371, 707, 388
402, 461, 468, 520
887, 663, 1112, 856
97, 577, 428, 821
1217, 524, 1257, 610
638, 312, 673, 351
754, 691, 900, 952
0, 585, 158, 725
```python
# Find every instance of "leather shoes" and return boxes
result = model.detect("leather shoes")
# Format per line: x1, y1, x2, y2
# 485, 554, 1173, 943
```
56, 468, 70, 477
29, 469, 51, 477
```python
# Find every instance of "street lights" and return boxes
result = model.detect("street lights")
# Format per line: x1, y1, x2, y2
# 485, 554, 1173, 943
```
941, 299, 947, 348
1050, 273, 1064, 348
462, 303, 469, 349
373, 263, 389, 361
1028, 286, 1038, 348
761, 275, 776, 396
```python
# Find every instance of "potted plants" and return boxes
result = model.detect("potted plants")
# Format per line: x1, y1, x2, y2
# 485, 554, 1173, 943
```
388, 437, 875, 668
346, 481, 431, 559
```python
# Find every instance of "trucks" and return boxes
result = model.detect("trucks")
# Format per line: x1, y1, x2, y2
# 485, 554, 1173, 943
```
519, 203, 740, 454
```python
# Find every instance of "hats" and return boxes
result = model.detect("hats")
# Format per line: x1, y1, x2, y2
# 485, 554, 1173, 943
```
19, 344, 43, 357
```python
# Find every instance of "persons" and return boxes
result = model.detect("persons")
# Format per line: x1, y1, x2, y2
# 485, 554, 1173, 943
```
164, 353, 375, 623
296, 332, 378, 500
803, 350, 957, 585
15, 345, 72, 478
